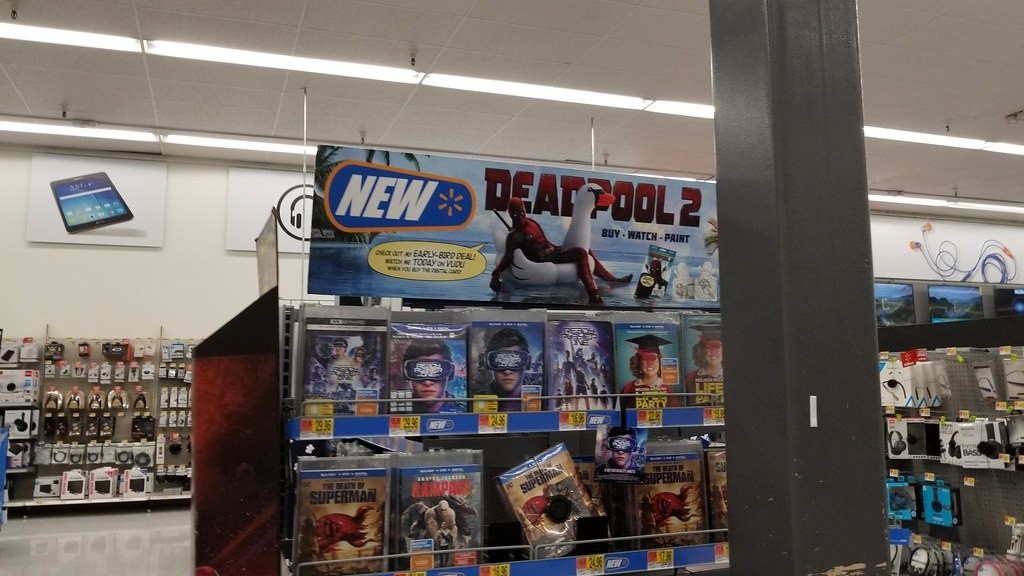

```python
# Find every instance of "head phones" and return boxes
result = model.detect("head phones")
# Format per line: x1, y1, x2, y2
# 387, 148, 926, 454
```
907, 545, 949, 576
889, 431, 905, 456
948, 431, 961, 459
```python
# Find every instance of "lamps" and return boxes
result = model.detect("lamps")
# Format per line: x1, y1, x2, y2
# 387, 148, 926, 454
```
985, 142, 1023, 157
142, 39, 425, 85
0, 114, 160, 143
159, 128, 318, 155
949, 198, 1024, 214
421, 73, 654, 111
868, 190, 949, 207
0, 23, 141, 53
863, 125, 985, 149
644, 99, 715, 120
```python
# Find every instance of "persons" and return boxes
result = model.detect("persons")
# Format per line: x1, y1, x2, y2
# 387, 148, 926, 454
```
434, 521, 456, 568
649, 259, 669, 289
314, 319, 727, 415
595, 425, 645, 483
489, 196, 634, 308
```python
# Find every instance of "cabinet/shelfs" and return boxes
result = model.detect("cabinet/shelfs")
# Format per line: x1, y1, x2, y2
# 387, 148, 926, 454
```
283, 406, 730, 576
1, 322, 205, 521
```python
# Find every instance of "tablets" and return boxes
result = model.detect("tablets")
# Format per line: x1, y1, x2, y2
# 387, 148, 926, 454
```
50, 172, 134, 234
